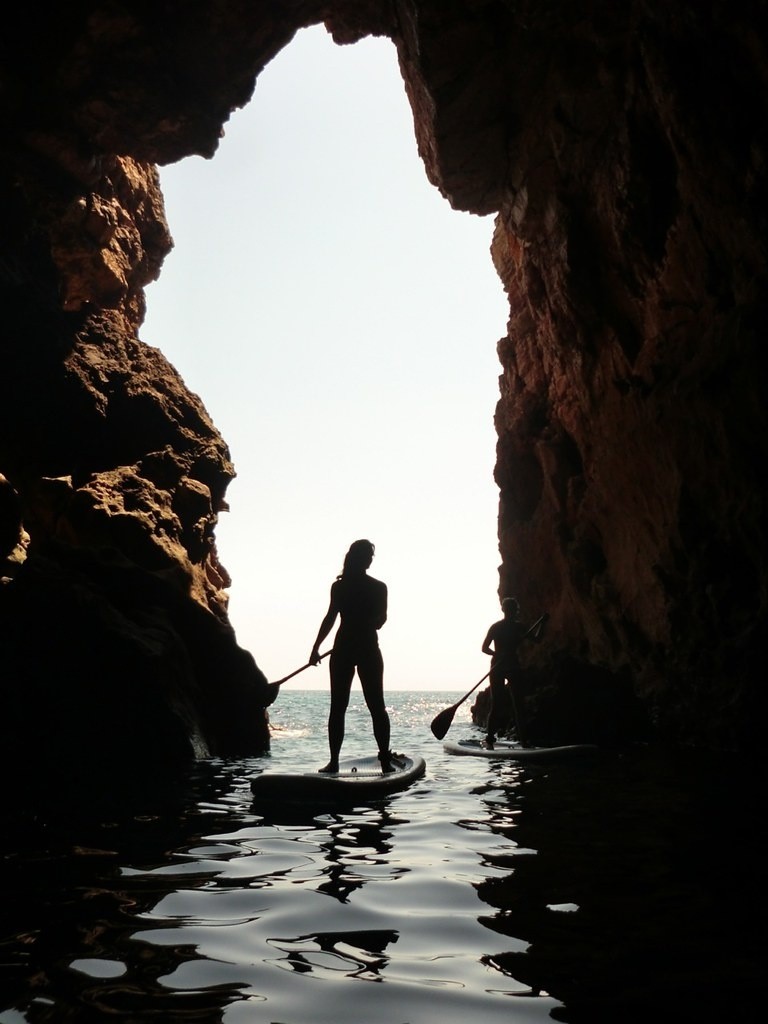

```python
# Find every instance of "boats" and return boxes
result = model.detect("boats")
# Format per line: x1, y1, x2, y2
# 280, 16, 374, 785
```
441, 732, 599, 760
250, 749, 426, 811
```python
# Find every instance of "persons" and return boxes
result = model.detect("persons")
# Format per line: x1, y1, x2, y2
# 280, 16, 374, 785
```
310, 539, 399, 777
482, 597, 549, 748
